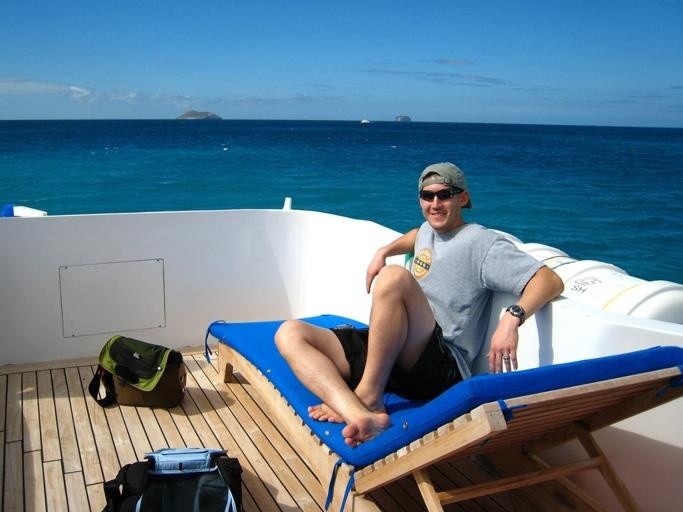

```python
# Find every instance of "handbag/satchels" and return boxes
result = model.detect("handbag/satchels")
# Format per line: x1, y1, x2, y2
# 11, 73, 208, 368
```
99, 335, 186, 408
122, 448, 245, 512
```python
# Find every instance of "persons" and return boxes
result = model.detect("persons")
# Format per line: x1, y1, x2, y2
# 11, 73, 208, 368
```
274, 162, 563, 448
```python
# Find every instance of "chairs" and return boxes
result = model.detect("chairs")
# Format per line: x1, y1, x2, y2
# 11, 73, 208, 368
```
209, 312, 683, 511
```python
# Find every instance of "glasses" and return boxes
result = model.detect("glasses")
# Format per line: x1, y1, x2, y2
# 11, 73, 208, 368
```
420, 188, 461, 201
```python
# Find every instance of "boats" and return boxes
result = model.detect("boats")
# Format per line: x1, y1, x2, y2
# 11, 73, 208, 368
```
361, 119, 370, 123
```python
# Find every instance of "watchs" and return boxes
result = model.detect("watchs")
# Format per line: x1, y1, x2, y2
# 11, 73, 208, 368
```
506, 304, 526, 326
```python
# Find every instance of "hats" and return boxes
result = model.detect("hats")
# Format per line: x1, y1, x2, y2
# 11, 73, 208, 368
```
418, 162, 471, 208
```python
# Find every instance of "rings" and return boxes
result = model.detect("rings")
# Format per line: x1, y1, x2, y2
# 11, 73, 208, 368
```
503, 356, 510, 360
